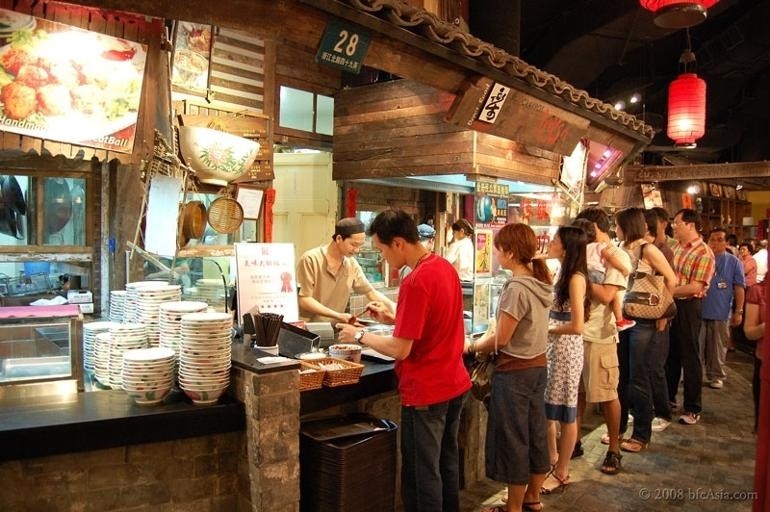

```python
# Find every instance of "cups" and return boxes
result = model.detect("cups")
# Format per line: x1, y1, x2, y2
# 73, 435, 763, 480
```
254, 344, 279, 355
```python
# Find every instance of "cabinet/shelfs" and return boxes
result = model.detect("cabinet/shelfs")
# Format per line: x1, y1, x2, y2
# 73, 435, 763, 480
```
684, 180, 752, 245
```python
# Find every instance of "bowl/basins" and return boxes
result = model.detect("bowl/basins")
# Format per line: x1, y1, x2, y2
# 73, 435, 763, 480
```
178, 126, 262, 188
328, 344, 362, 363
83, 320, 116, 377
121, 346, 176, 405
94, 332, 111, 387
157, 300, 208, 391
134, 284, 183, 348
177, 311, 234, 404
111, 324, 148, 391
122, 279, 170, 323
110, 289, 126, 324
174, 49, 209, 84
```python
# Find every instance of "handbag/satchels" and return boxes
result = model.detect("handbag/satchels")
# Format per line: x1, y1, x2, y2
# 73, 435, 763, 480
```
623, 270, 678, 321
468, 357, 493, 400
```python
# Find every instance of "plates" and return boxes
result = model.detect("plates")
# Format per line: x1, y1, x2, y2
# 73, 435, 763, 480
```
196, 279, 230, 302
182, 286, 198, 302
0, 7, 38, 37
293, 350, 328, 359
0, 29, 144, 143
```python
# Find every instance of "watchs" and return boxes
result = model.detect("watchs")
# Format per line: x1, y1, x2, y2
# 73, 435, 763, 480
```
353, 330, 367, 343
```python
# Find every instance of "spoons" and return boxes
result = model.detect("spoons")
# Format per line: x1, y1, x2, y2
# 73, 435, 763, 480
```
348, 303, 377, 325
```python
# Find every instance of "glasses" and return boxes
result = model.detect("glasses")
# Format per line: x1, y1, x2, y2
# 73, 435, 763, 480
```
670, 221, 689, 228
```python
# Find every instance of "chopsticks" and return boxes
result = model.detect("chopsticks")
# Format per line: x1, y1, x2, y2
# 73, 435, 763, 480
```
253, 311, 285, 347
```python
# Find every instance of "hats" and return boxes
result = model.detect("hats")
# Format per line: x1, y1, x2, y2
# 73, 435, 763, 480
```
415, 222, 437, 239
456, 217, 475, 235
334, 216, 366, 235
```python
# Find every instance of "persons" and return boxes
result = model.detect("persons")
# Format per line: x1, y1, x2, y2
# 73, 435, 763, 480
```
145, 253, 201, 294
541, 207, 769, 512
396, 223, 435, 285
443, 219, 475, 282
298, 216, 396, 326
460, 223, 554, 512
335, 206, 473, 512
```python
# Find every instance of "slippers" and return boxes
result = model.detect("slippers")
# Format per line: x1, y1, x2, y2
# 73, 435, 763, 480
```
625, 414, 634, 423
601, 433, 624, 444
650, 417, 673, 431
619, 438, 649, 452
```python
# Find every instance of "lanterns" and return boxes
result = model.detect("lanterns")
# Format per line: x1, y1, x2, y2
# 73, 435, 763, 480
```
640, 0, 718, 28
667, 48, 708, 151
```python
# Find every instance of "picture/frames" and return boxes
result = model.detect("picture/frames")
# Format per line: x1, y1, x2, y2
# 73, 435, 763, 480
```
170, 18, 215, 98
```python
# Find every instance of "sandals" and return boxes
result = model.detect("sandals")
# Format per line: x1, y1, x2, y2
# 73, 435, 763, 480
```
501, 492, 544, 512
481, 505, 525, 512
571, 440, 584, 459
600, 451, 622, 475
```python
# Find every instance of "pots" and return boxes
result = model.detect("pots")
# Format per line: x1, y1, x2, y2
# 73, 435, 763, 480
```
43, 175, 72, 236
0, 197, 20, 241
1, 175, 26, 215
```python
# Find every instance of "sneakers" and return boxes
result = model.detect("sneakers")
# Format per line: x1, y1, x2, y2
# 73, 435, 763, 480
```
679, 411, 702, 425
710, 377, 723, 390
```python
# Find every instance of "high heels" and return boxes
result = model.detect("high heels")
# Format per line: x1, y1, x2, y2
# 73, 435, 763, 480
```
539, 469, 572, 498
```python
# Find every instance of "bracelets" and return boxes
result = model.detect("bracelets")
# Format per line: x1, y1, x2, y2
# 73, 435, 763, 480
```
468, 340, 477, 355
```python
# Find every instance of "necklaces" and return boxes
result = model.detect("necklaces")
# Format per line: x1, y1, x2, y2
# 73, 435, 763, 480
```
413, 249, 431, 269
